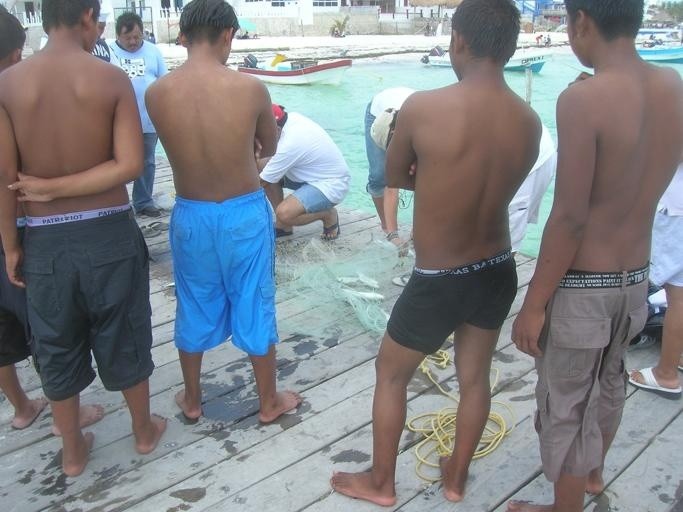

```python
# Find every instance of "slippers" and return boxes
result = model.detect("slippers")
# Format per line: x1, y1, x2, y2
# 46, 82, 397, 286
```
274, 227, 293, 236
627, 334, 655, 352
627, 367, 682, 397
323, 213, 339, 240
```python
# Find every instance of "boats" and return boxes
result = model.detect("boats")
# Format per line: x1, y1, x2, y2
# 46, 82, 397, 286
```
236, 53, 353, 86
420, 43, 548, 74
633, 39, 683, 63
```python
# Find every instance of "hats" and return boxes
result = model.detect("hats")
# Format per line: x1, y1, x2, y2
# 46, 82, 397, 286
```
272, 104, 285, 125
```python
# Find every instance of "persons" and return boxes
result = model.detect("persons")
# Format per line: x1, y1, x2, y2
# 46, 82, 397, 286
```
504, 0, 682, 512
627, 169, 683, 393
90, 12, 168, 216
2, 0, 170, 479
366, 87, 417, 247
507, 125, 557, 258
142, 1, 305, 423
327, 0, 544, 509
259, 104, 352, 240
536, 34, 552, 45
0, 1, 107, 439
25, 0, 36, 23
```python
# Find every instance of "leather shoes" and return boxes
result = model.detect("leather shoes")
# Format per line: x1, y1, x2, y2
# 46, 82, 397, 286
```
139, 206, 160, 217
